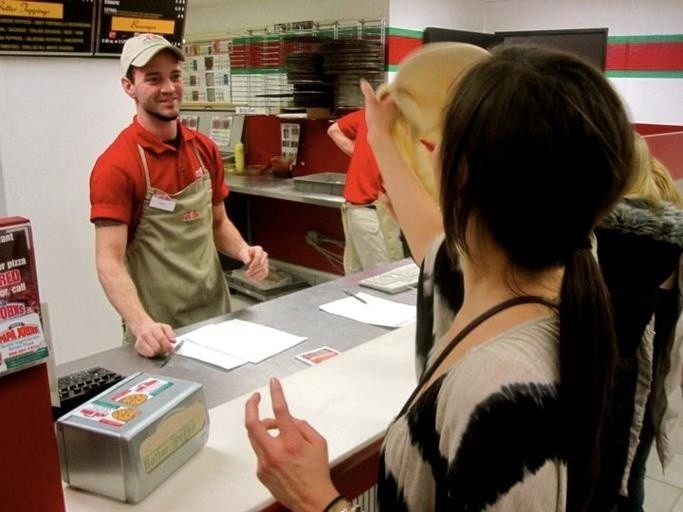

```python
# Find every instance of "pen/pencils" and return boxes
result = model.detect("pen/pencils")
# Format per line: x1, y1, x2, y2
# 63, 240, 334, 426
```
343, 290, 367, 304
160, 340, 185, 368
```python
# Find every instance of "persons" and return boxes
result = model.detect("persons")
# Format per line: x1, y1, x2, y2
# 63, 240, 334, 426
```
325, 81, 403, 274
592, 132, 682, 497
243, 43, 639, 512
88, 33, 269, 357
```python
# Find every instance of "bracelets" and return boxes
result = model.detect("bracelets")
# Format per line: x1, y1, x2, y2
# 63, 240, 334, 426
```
322, 492, 348, 512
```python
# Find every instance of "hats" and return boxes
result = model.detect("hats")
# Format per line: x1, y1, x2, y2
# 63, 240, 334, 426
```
119, 31, 186, 79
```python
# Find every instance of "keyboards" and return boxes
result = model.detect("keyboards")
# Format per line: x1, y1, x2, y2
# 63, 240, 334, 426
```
52, 359, 124, 421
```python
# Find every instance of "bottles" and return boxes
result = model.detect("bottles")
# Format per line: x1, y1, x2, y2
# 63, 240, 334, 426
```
234, 144, 245, 169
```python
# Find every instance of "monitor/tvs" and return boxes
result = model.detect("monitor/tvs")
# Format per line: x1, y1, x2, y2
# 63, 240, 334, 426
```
497, 28, 607, 72
